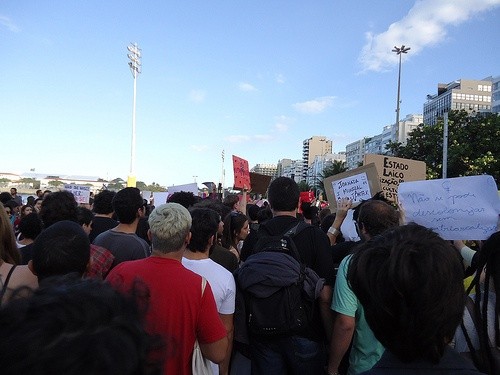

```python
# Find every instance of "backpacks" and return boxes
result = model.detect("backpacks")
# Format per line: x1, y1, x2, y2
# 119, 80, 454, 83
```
234, 220, 323, 336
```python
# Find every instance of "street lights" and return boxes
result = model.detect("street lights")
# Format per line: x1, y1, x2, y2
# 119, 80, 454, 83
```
391, 45, 410, 121
221, 149, 225, 202
127, 42, 140, 187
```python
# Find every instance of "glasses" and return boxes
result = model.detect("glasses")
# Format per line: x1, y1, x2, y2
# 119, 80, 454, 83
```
6, 211, 13, 215
231, 211, 243, 217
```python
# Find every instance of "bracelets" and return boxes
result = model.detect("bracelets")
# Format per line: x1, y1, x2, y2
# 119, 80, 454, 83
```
328, 227, 340, 237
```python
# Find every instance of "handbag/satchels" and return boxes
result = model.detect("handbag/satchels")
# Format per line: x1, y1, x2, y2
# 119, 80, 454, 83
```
470, 347, 500, 375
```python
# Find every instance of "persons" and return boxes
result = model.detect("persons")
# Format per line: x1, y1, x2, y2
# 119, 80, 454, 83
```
0, 272, 166, 375
181, 208, 237, 375
347, 222, 500, 374
105, 202, 229, 375
0, 188, 500, 274
240, 176, 333, 375
327, 199, 400, 374
28, 220, 91, 287
92, 187, 151, 266
0, 201, 39, 304
